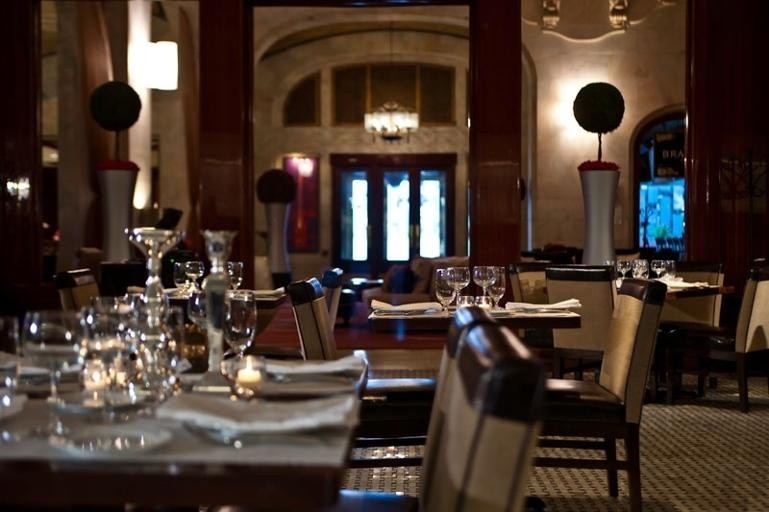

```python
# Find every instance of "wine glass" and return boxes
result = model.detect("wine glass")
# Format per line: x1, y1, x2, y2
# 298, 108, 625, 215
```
184, 259, 205, 292
222, 289, 258, 357
224, 259, 244, 295
433, 262, 508, 315
615, 256, 676, 284
23, 312, 89, 409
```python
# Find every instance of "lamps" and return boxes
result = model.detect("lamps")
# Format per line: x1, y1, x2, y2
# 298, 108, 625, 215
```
141, 41, 178, 90
364, 21, 420, 145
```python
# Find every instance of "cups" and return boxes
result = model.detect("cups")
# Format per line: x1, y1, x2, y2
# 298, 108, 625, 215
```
172, 262, 188, 287
455, 294, 495, 313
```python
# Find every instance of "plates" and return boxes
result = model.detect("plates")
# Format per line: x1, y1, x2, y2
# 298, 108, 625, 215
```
62, 421, 174, 461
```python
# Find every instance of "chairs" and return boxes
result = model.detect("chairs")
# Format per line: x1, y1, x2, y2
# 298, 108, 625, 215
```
652, 257, 768, 414
51, 255, 668, 512
